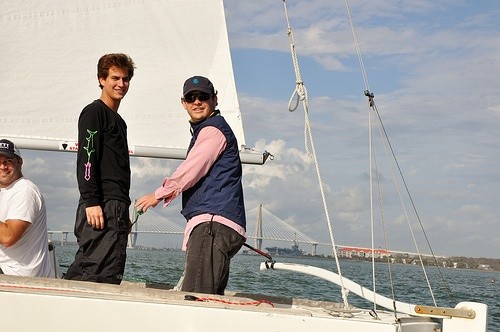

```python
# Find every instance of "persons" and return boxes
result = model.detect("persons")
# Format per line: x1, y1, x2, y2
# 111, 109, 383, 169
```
134, 76, 247, 295
0, 139, 52, 277
63, 53, 137, 284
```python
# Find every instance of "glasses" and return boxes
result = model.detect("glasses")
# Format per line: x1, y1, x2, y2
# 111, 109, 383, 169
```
185, 92, 210, 102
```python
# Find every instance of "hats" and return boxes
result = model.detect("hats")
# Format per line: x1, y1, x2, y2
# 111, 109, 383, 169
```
0, 139, 20, 158
183, 76, 214, 95
